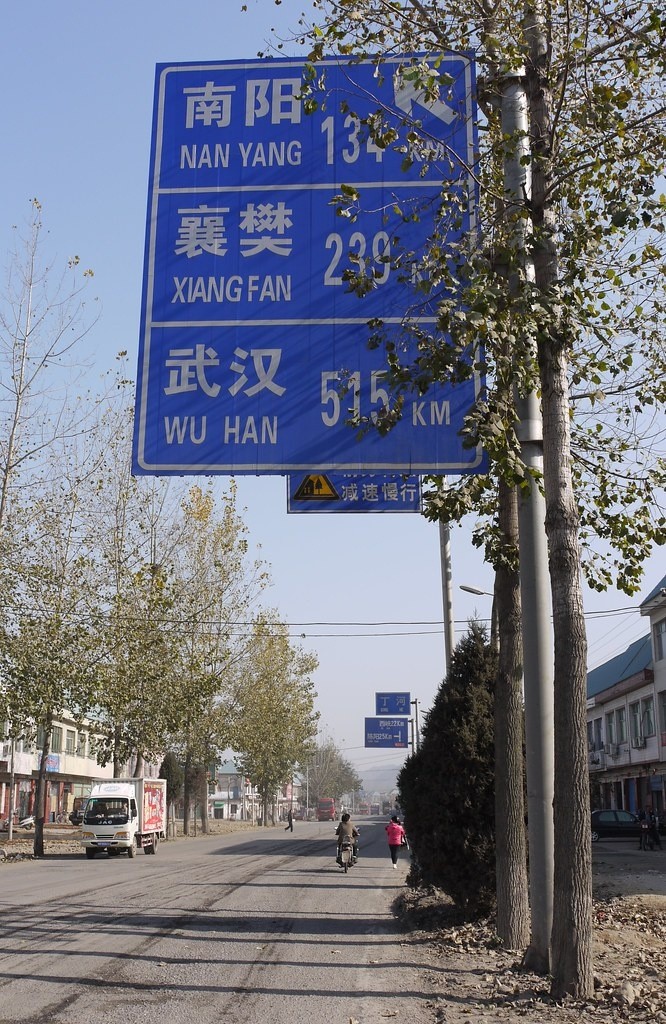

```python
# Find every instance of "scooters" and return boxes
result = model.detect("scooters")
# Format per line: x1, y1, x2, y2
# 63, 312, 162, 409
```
3, 806, 34, 831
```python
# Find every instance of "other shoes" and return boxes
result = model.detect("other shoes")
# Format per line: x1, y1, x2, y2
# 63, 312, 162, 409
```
393, 864, 398, 869
353, 857, 357, 863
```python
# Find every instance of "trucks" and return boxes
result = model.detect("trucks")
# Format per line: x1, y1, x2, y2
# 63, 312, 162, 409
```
81, 777, 166, 858
317, 797, 342, 820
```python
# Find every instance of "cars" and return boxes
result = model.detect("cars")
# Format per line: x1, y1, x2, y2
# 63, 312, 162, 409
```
590, 809, 642, 842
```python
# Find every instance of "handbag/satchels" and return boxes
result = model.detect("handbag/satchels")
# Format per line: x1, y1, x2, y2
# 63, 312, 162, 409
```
401, 836, 405, 843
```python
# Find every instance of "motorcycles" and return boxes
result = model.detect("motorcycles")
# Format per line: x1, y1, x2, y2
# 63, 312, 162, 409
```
632, 815, 656, 851
334, 826, 360, 873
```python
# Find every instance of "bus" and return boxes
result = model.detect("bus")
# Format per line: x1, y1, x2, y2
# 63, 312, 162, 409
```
359, 802, 369, 815
371, 801, 393, 815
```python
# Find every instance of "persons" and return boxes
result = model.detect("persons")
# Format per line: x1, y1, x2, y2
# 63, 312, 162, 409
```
335, 813, 360, 859
284, 809, 294, 832
385, 816, 404, 869
121, 802, 132, 815
